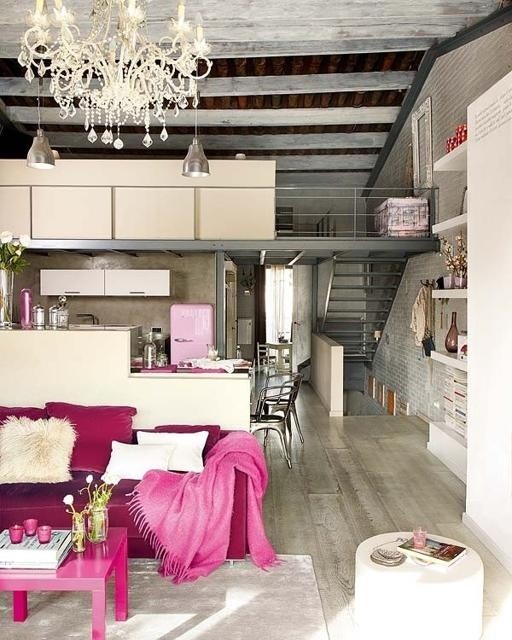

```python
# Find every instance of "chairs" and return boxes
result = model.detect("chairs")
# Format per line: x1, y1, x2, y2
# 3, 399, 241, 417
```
251, 333, 308, 474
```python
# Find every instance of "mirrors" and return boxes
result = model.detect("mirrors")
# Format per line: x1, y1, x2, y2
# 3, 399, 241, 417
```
409, 96, 435, 194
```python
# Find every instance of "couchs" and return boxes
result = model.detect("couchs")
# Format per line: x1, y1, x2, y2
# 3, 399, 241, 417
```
0, 399, 260, 563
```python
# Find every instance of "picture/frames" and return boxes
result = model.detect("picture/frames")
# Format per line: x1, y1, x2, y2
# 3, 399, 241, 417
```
367, 374, 412, 417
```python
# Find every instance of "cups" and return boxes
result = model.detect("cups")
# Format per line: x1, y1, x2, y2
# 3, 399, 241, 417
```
156, 351, 165, 367
412, 527, 427, 550
445, 137, 454, 154
454, 124, 467, 147
9, 525, 24, 544
36, 525, 52, 543
23, 519, 39, 537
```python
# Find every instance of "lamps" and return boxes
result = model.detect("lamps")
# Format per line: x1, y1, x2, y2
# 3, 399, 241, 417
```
26, 69, 56, 175
179, 76, 213, 181
16, 0, 219, 147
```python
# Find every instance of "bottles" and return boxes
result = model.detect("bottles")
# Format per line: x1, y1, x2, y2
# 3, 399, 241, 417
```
206, 343, 217, 361
21, 287, 33, 327
445, 310, 459, 353
32, 303, 45, 327
57, 303, 70, 329
49, 307, 59, 327
144, 334, 154, 369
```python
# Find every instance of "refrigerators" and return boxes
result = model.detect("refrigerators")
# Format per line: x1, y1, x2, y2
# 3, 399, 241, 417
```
170, 304, 215, 366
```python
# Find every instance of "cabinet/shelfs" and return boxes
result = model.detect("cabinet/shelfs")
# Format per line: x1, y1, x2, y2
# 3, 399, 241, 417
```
103, 268, 174, 299
0, 154, 280, 244
427, 139, 468, 487
40, 267, 105, 299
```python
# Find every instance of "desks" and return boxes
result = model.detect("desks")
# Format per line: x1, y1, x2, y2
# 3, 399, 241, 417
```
2, 524, 133, 640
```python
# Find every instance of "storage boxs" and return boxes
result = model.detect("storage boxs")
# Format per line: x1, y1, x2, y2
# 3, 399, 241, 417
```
372, 194, 431, 237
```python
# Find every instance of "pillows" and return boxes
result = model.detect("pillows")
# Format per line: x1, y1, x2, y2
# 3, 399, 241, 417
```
0, 402, 227, 493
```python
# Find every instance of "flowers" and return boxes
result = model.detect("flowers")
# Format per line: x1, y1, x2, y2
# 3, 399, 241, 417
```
56, 487, 92, 546
82, 464, 119, 538
437, 230, 467, 278
0, 227, 35, 318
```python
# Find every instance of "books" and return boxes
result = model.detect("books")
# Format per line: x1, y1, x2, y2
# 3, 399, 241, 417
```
396, 537, 467, 568
139, 364, 176, 374
130, 357, 157, 369
0, 528, 86, 570
413, 555, 431, 567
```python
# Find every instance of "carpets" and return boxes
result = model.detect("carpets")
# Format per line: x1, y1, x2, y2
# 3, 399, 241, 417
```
2, 546, 330, 640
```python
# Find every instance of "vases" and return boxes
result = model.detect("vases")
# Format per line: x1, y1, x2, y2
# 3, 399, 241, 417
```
0, 267, 15, 331
441, 273, 452, 288
452, 268, 467, 291
85, 506, 112, 546
67, 515, 88, 554
443, 309, 459, 355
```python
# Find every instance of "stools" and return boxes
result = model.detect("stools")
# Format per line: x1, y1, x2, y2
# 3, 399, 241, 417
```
354, 527, 486, 640
